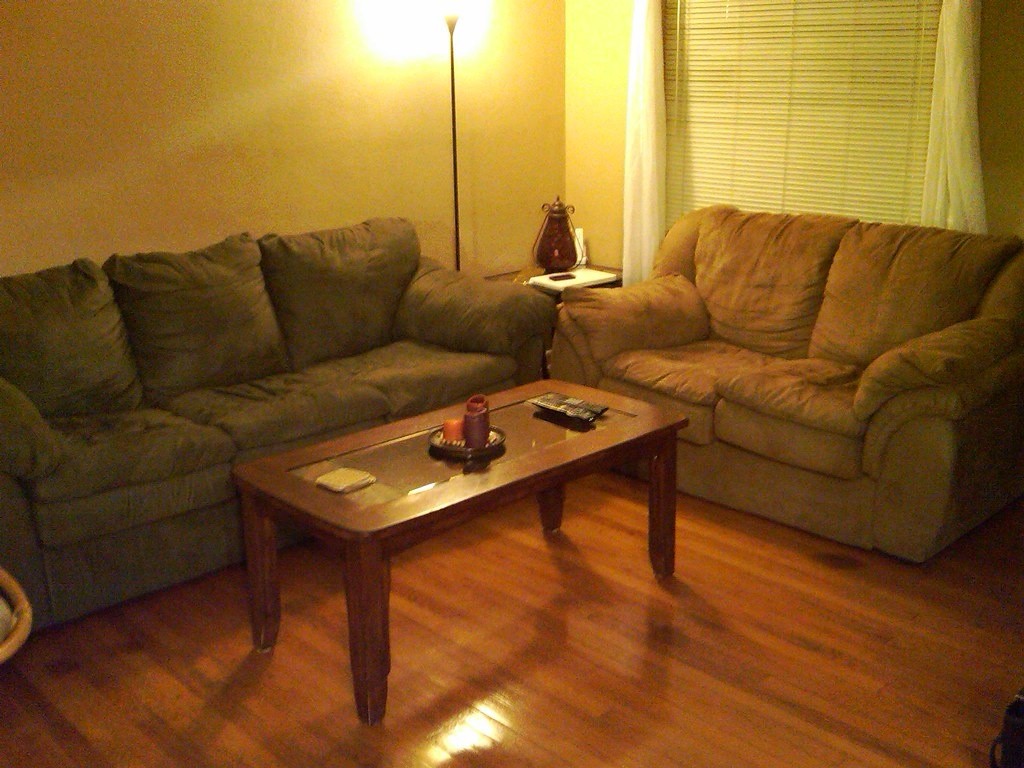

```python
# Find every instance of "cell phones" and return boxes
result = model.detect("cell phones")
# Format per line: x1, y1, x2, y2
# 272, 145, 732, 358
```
549, 273, 576, 281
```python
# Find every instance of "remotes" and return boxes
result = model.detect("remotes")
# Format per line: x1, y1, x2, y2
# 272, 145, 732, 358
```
532, 392, 610, 421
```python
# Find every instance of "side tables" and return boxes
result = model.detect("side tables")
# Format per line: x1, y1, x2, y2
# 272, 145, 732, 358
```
487, 261, 623, 302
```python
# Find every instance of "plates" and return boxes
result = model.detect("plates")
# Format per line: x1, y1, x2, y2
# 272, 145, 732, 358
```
427, 423, 505, 458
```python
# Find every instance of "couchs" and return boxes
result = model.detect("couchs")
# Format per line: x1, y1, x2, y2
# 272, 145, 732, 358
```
545, 203, 1024, 565
0, 213, 561, 632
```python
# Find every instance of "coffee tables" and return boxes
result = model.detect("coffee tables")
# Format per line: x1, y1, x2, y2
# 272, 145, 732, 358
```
232, 377, 689, 728
